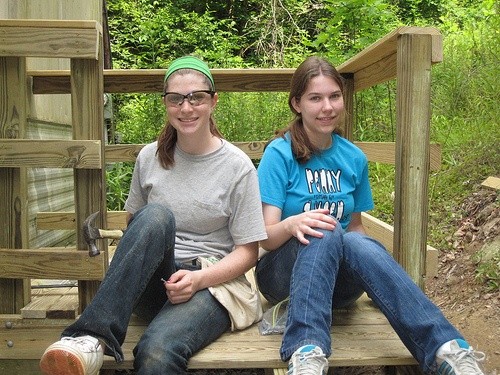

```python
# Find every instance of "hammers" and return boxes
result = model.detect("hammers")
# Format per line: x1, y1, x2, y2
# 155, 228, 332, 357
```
82, 210, 126, 257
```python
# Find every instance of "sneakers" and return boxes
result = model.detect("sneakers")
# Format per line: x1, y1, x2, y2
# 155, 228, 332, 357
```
434, 338, 487, 375
39, 334, 105, 375
287, 345, 329, 375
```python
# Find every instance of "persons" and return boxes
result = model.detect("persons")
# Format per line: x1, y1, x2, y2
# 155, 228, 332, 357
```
254, 56, 485, 375
40, 56, 268, 375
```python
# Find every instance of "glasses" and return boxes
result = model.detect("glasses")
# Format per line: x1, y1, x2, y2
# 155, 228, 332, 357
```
161, 90, 215, 107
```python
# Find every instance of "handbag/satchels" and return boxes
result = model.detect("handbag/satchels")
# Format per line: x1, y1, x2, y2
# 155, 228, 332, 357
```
197, 254, 264, 332
